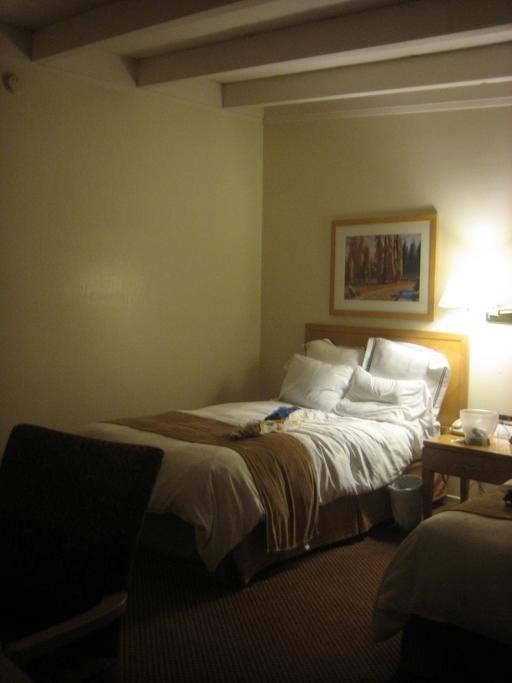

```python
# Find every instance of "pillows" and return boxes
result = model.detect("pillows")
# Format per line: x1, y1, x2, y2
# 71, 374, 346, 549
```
336, 366, 428, 423
302, 338, 365, 366
278, 353, 354, 411
361, 337, 446, 422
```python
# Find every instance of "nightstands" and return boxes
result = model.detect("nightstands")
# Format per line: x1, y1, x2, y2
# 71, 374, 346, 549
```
421, 413, 512, 520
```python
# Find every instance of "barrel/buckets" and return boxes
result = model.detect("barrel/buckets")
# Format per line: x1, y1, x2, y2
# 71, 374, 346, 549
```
460, 408, 498, 447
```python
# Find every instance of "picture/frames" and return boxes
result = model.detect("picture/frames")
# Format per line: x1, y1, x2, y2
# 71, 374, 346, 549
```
329, 213, 439, 320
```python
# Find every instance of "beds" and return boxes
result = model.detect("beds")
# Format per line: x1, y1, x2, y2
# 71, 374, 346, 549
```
72, 323, 471, 605
374, 478, 512, 683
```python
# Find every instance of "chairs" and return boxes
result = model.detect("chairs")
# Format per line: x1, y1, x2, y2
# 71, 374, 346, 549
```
0, 424, 166, 683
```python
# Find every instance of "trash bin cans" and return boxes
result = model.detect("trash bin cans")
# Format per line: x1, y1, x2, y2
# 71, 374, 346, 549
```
388, 473, 423, 531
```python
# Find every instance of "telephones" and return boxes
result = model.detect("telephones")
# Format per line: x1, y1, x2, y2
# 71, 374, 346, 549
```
449, 418, 465, 435
